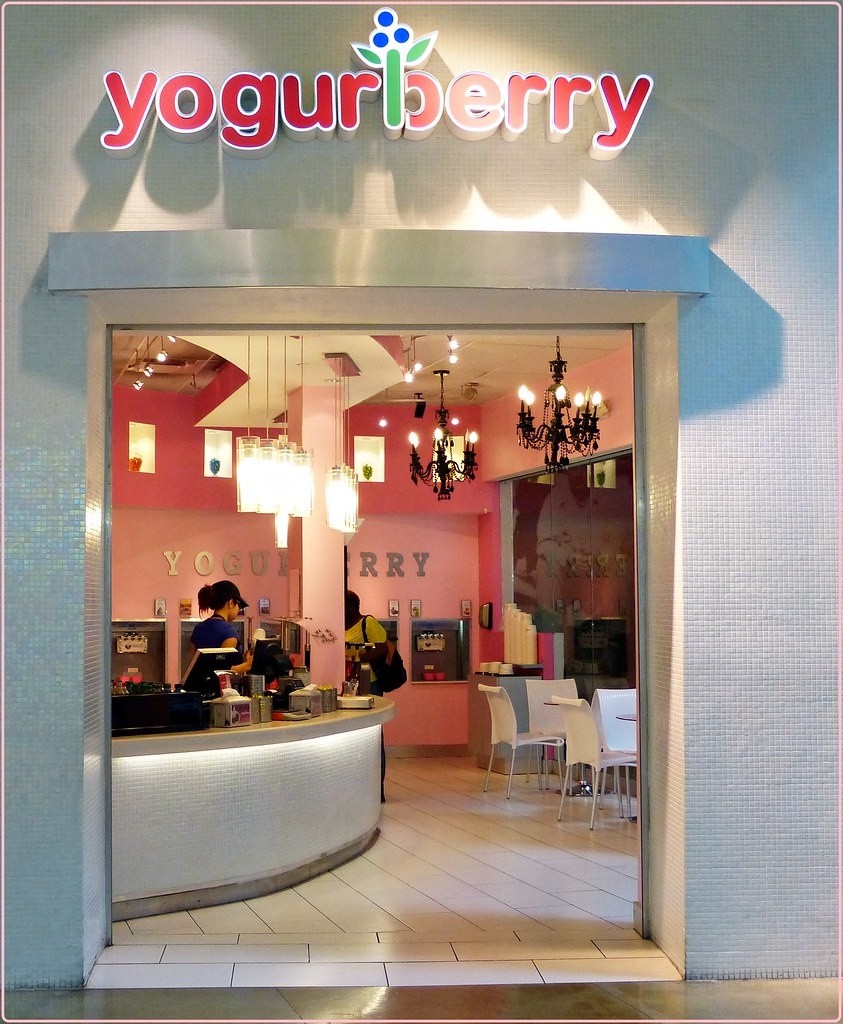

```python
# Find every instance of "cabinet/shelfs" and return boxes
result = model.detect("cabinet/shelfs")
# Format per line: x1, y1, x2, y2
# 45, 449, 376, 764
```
467, 673, 543, 775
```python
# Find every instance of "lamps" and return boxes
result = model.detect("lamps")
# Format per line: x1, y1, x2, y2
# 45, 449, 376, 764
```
325, 353, 361, 533
447, 335, 459, 364
516, 336, 602, 474
404, 335, 422, 382
409, 369, 478, 500
414, 392, 426, 419
143, 335, 154, 378
235, 335, 312, 549
157, 336, 169, 362
133, 350, 143, 391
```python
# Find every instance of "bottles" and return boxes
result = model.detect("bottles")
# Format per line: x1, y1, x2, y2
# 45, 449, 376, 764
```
152, 684, 172, 693
359, 665, 370, 696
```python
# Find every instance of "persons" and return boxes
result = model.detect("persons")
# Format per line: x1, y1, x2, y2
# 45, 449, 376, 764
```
347, 589, 387, 801
189, 581, 253, 675
535, 465, 634, 617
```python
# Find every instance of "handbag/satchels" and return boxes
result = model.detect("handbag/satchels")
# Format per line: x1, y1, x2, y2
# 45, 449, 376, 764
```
361, 615, 407, 693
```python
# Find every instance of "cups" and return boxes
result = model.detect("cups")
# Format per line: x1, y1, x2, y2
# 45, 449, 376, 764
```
503, 603, 538, 665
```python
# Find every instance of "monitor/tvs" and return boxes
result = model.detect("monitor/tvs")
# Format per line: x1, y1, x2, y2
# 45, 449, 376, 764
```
249, 637, 294, 684
180, 647, 238, 693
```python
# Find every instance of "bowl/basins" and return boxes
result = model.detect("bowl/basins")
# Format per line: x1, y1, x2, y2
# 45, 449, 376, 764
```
499, 664, 513, 674
479, 663, 490, 672
489, 662, 502, 673
433, 672, 446, 681
423, 673, 434, 681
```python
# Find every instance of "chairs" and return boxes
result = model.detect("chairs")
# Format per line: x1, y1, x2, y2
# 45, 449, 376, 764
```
551, 696, 639, 830
524, 678, 588, 789
589, 689, 639, 812
478, 683, 566, 800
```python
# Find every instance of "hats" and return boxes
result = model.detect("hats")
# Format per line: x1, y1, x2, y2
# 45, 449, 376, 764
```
212, 581, 249, 609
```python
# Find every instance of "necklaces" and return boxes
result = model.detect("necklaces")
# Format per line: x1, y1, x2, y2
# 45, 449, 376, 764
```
211, 614, 225, 622
